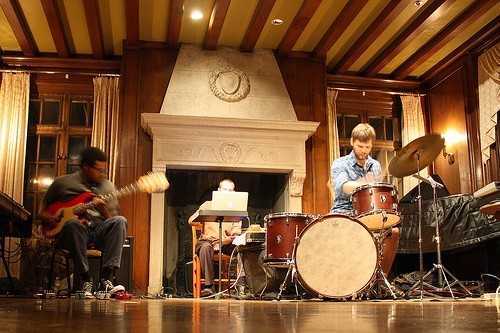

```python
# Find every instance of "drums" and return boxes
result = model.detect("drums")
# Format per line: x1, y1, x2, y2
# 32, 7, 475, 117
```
263, 213, 317, 269
351, 182, 403, 230
294, 213, 379, 297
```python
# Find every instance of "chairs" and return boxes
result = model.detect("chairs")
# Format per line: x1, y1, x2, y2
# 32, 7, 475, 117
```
46, 244, 104, 298
190, 226, 244, 298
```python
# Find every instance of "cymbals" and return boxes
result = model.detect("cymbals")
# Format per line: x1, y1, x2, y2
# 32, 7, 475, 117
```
388, 131, 445, 179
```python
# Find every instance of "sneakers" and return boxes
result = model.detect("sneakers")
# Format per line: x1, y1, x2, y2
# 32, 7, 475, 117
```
76, 280, 94, 298
98, 279, 125, 294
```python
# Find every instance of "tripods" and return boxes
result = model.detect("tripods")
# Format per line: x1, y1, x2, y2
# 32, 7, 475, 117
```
365, 152, 473, 300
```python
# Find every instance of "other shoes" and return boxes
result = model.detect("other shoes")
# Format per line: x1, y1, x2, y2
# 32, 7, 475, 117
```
236, 291, 246, 300
201, 288, 213, 296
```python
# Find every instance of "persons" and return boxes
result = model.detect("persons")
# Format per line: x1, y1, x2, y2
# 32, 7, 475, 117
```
37, 146, 128, 299
187, 179, 246, 297
330, 123, 401, 300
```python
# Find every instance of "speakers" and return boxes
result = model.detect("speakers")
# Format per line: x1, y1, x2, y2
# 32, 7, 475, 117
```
73, 235, 134, 295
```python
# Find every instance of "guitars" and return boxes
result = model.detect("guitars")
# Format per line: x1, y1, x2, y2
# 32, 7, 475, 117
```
41, 171, 168, 239
